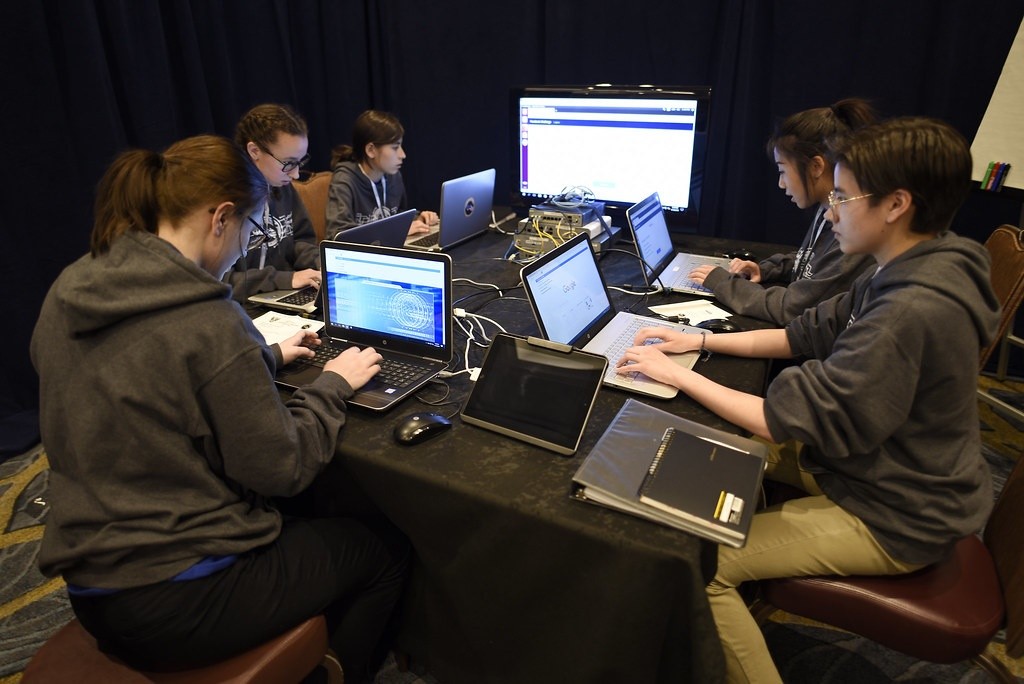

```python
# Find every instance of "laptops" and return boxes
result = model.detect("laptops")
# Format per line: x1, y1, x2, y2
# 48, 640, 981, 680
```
274, 240, 453, 411
247, 207, 416, 317
626, 192, 747, 297
520, 231, 713, 398
402, 168, 497, 252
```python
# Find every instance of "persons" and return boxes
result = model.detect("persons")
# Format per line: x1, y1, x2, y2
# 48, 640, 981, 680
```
30, 134, 414, 684
687, 99, 879, 327
326, 111, 438, 240
219, 104, 322, 303
614, 115, 1001, 684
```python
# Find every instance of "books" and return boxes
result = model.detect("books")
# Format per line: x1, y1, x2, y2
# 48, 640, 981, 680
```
636, 427, 765, 540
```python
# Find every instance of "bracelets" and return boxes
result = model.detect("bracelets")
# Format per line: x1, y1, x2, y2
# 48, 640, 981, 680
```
699, 332, 705, 354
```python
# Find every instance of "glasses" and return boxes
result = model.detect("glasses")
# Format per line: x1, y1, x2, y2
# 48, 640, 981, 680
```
828, 188, 873, 222
207, 206, 271, 251
254, 140, 311, 172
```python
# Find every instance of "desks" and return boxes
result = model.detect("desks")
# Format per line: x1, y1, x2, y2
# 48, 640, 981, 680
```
245, 208, 801, 684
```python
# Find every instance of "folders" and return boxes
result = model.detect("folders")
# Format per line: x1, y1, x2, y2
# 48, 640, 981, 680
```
569, 398, 768, 548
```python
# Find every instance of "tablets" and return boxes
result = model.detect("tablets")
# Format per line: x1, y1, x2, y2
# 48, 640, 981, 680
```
460, 333, 608, 455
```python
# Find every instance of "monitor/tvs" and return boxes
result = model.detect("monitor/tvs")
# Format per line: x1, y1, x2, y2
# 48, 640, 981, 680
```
508, 83, 712, 232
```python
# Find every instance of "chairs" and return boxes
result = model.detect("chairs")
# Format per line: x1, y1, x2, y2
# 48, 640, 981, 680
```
735, 448, 1023, 684
980, 223, 1024, 370
291, 171, 332, 243
22, 613, 344, 684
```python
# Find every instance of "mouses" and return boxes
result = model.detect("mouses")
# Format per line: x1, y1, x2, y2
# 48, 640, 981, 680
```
722, 249, 753, 261
393, 411, 453, 445
696, 319, 742, 334
